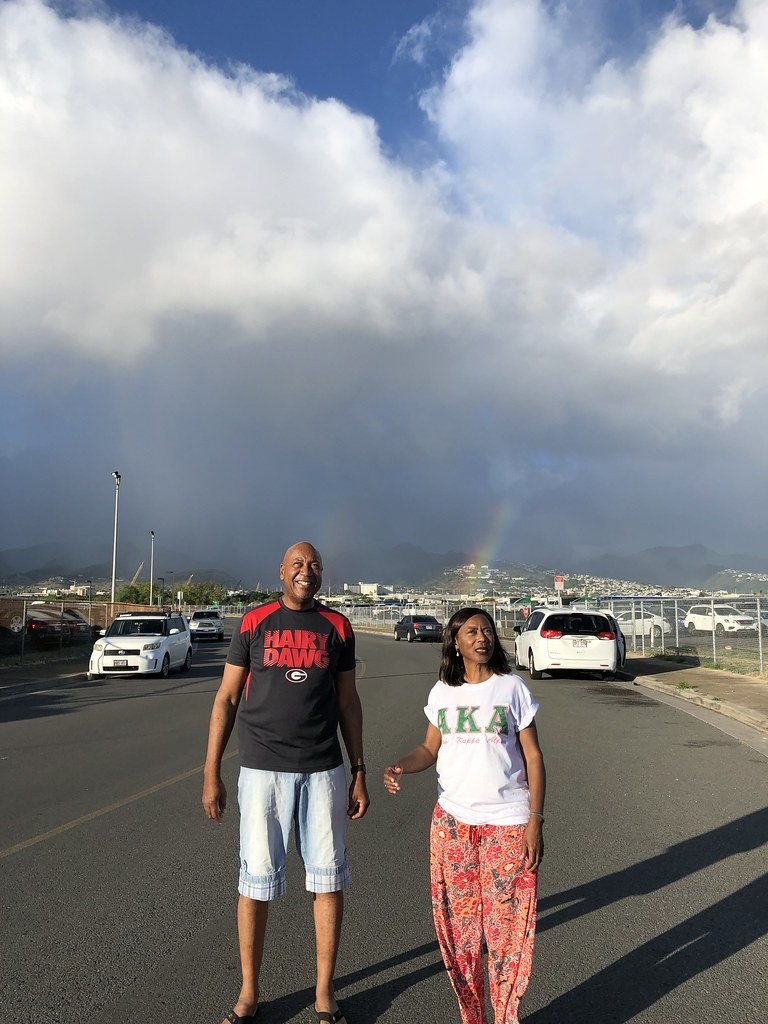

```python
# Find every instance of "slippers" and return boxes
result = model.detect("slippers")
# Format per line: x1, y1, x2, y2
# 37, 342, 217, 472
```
221, 1004, 258, 1024
313, 1002, 347, 1024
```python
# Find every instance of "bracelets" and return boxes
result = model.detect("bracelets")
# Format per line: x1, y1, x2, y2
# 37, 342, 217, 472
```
529, 812, 545, 822
350, 765, 366, 774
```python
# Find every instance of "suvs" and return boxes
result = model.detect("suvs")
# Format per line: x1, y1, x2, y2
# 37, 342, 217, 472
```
89, 611, 193, 679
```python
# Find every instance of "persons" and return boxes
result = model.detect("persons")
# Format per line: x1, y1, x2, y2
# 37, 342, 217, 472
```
201, 540, 370, 1023
383, 607, 546, 1024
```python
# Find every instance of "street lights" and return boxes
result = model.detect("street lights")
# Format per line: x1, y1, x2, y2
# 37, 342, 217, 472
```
158, 578, 164, 605
171, 572, 174, 609
87, 580, 91, 601
110, 471, 121, 617
150, 531, 155, 606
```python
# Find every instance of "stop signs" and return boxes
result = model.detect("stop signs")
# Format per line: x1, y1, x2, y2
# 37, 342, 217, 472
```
524, 608, 528, 617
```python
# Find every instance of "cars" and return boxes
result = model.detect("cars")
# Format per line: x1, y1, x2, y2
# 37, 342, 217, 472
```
0, 606, 92, 646
725, 602, 768, 629
186, 610, 225, 642
634, 606, 686, 623
393, 615, 443, 643
378, 611, 404, 620
512, 608, 626, 681
683, 604, 757, 637
614, 611, 672, 639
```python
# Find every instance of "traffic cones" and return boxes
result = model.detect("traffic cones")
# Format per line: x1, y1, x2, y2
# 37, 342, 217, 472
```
502, 628, 506, 637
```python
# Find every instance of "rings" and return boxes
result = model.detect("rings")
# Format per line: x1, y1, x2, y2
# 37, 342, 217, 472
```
385, 784, 387, 787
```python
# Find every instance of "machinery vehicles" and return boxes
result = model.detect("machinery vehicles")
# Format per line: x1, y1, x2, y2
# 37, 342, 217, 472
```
129, 561, 144, 586
256, 582, 260, 592
185, 574, 193, 585
